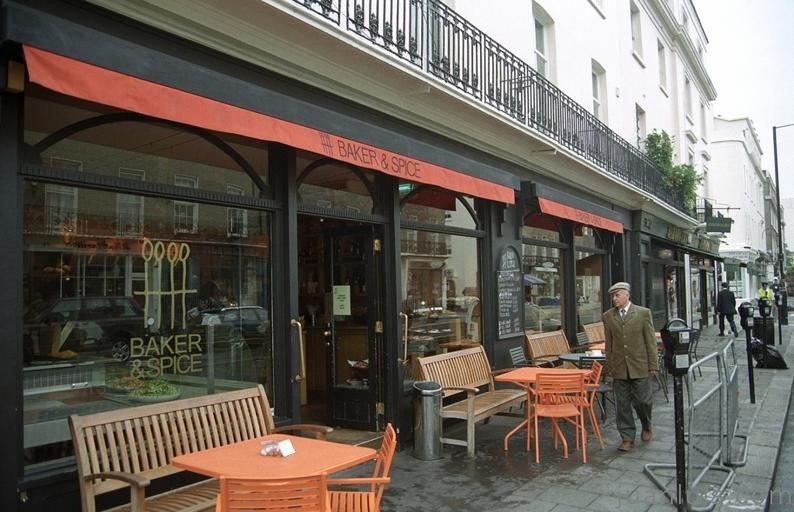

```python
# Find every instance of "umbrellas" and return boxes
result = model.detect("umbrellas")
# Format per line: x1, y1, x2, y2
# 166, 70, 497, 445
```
524, 273, 548, 286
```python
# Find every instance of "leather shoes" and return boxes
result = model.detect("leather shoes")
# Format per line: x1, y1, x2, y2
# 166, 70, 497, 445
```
617, 442, 634, 451
641, 427, 652, 441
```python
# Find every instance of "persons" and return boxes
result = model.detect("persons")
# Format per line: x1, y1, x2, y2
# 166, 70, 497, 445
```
773, 279, 780, 293
196, 281, 224, 312
759, 281, 773, 307
602, 281, 659, 452
299, 264, 324, 320
716, 282, 739, 337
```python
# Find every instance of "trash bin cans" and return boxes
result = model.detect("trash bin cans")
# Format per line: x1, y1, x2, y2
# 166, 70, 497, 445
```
753, 317, 774, 346
413, 382, 442, 460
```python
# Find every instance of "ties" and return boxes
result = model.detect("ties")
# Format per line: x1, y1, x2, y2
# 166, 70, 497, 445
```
621, 309, 626, 322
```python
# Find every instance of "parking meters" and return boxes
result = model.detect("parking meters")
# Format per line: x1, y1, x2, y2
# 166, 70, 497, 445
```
659, 318, 692, 511
774, 293, 785, 346
756, 297, 773, 368
738, 300, 758, 404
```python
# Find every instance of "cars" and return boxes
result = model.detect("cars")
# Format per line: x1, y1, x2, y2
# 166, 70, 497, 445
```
536, 296, 593, 308
180, 303, 269, 351
407, 294, 479, 317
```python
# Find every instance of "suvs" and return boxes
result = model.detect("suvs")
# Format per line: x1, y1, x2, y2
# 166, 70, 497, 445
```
24, 295, 146, 367
464, 299, 561, 343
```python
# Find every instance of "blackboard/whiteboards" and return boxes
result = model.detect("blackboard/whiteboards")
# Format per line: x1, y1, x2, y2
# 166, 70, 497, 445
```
497, 245, 525, 340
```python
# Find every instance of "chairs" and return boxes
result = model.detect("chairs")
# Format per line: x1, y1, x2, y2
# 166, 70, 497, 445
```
59, 312, 702, 511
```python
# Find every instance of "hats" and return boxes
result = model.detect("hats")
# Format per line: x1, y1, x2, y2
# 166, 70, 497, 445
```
608, 282, 631, 294
